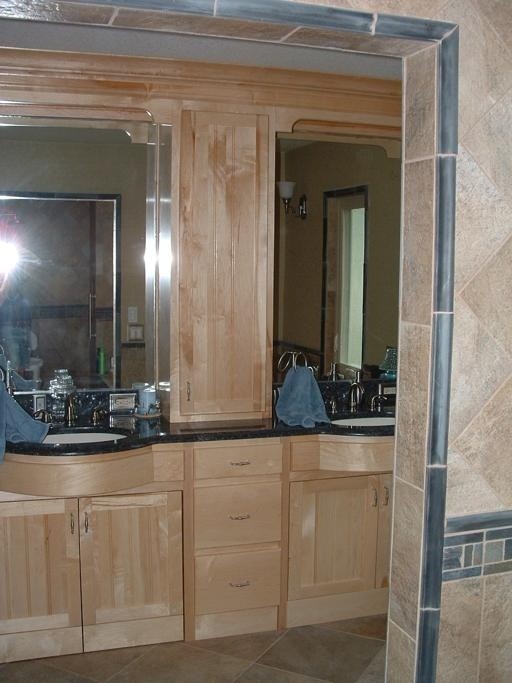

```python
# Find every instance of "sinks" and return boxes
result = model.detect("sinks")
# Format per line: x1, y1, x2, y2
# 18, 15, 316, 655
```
327, 413, 398, 427
35, 427, 133, 444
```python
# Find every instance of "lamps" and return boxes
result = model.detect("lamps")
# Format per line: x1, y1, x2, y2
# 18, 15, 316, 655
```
275, 180, 307, 219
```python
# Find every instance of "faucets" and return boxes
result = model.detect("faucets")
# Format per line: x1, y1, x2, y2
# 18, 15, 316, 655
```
66, 394, 78, 427
348, 382, 366, 411
371, 395, 388, 411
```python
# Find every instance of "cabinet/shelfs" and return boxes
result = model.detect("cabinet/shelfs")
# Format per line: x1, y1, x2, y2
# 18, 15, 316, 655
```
283, 434, 392, 629
2, 447, 184, 662
183, 435, 290, 642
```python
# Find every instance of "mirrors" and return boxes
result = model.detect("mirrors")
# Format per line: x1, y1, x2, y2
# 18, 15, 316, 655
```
0, 115, 175, 394
271, 116, 403, 385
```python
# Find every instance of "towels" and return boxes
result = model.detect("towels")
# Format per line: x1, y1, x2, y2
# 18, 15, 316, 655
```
0, 355, 34, 391
0, 380, 50, 465
275, 365, 331, 427
